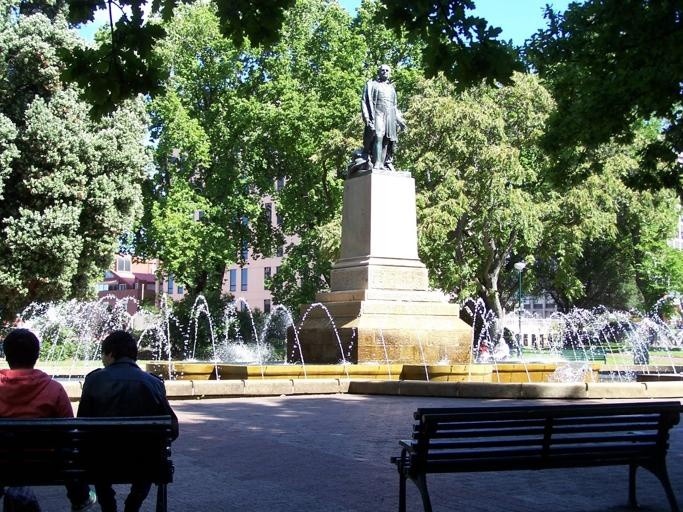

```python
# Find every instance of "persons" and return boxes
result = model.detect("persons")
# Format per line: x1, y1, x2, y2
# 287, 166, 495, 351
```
75, 331, 179, 512
494, 338, 509, 361
480, 340, 490, 362
0, 329, 96, 512
362, 64, 407, 170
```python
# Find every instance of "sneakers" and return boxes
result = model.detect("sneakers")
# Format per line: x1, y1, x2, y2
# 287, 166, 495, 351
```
70, 491, 97, 511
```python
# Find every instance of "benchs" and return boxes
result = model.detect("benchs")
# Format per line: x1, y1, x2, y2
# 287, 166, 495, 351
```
0, 418, 180, 511
387, 401, 681, 511
